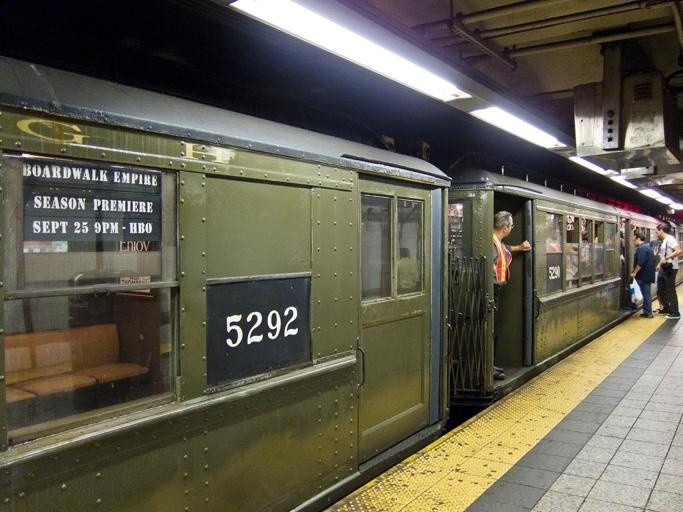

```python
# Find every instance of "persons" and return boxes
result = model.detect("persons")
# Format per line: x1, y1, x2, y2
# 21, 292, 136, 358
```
396, 246, 420, 293
630, 235, 654, 318
655, 223, 682, 322
547, 228, 626, 286
491, 209, 531, 381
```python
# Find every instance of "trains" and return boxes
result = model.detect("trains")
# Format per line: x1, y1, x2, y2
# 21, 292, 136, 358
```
0, 56, 683, 512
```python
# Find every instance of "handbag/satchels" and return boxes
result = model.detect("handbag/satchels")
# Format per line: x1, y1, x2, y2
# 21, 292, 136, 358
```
661, 262, 673, 273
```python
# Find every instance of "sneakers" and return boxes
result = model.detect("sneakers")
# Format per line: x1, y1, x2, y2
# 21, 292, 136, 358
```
639, 308, 680, 320
492, 366, 506, 381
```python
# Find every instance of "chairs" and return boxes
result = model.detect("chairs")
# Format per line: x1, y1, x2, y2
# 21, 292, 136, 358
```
2, 322, 151, 422
